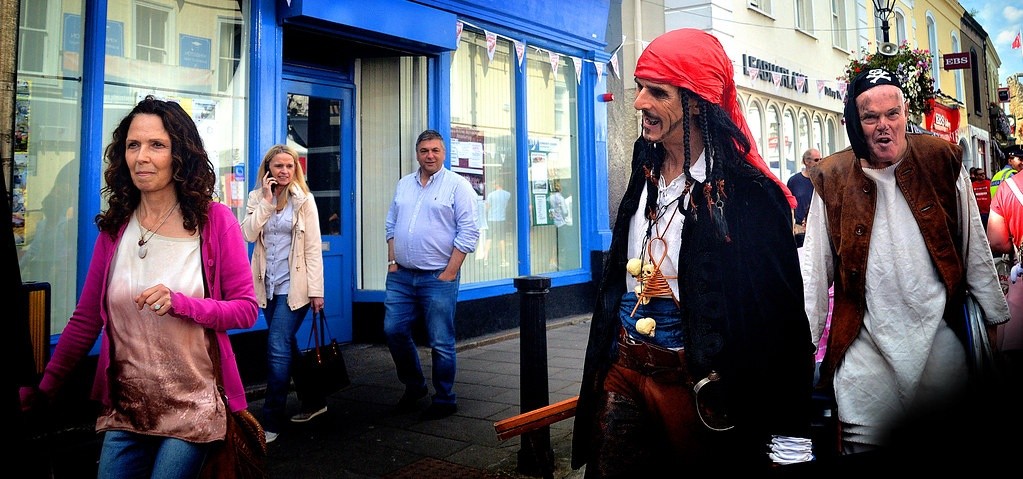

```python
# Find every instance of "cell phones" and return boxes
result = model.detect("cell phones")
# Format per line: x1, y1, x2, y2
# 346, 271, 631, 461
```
265, 163, 276, 188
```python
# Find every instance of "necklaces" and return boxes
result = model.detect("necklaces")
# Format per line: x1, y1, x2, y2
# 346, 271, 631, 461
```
137, 200, 178, 259
626, 156, 711, 337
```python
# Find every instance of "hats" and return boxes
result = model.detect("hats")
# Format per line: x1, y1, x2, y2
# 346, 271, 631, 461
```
1004, 144, 1023, 157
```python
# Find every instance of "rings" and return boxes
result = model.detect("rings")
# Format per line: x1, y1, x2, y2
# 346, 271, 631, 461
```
153, 303, 160, 310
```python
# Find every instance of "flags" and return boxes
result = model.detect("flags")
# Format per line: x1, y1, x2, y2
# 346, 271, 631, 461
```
1012, 29, 1022, 49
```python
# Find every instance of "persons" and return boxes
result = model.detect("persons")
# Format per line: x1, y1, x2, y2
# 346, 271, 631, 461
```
786, 68, 1023, 479
570, 28, 818, 479
0, 154, 37, 479
546, 178, 572, 270
240, 144, 327, 441
478, 177, 511, 268
37, 95, 259, 479
383, 131, 479, 415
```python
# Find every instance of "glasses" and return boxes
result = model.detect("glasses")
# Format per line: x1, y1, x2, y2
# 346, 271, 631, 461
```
807, 157, 823, 162
977, 173, 985, 176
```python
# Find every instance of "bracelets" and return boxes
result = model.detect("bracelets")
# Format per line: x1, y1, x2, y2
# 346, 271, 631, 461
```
388, 260, 397, 266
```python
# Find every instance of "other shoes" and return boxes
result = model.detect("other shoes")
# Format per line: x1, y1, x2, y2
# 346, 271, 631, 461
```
417, 402, 458, 422
291, 406, 327, 422
386, 389, 423, 416
265, 431, 280, 443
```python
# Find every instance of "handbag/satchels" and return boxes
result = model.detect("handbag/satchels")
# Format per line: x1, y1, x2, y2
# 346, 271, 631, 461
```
202, 407, 268, 479
304, 309, 352, 397
